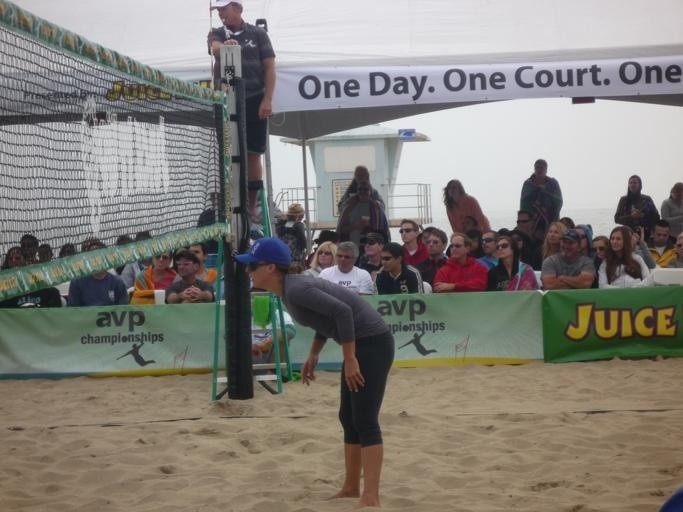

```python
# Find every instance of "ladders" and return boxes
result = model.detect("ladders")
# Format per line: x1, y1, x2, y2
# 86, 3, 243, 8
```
212, 179, 293, 403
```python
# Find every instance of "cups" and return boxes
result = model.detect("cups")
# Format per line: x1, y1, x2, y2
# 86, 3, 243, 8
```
533, 271, 541, 281
152, 289, 166, 305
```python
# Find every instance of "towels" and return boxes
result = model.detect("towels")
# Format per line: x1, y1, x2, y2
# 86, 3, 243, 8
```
287, 371, 302, 383
251, 295, 270, 327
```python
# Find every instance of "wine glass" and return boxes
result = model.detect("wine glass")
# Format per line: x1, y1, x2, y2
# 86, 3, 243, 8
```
250, 292, 274, 340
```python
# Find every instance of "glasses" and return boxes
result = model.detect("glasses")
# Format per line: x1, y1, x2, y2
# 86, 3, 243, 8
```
318, 250, 348, 260
247, 263, 267, 271
578, 233, 587, 240
155, 256, 169, 260
380, 256, 392, 261
398, 228, 461, 249
480, 239, 507, 250
516, 219, 530, 225
590, 247, 605, 252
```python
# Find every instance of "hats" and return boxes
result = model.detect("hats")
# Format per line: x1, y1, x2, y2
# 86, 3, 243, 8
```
235, 238, 293, 267
284, 204, 303, 216
559, 231, 581, 244
360, 233, 383, 244
508, 227, 531, 244
176, 251, 201, 268
209, 0, 242, 9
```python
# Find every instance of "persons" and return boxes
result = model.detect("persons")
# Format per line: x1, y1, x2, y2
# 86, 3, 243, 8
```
206, 0, 276, 208
235, 236, 395, 508
1, 229, 217, 307
192, 159, 683, 295
